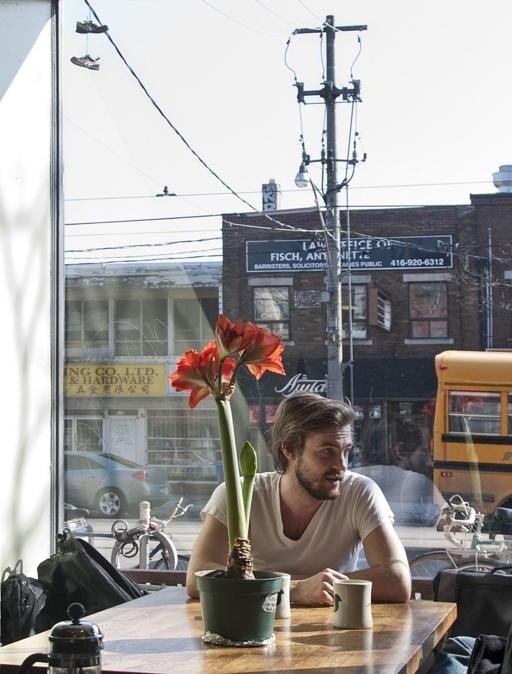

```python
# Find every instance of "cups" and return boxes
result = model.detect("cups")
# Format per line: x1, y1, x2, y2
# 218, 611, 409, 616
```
273, 572, 291, 619
332, 580, 373, 628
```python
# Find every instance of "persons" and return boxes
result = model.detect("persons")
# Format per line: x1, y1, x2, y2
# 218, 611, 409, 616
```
345, 418, 470, 577
185, 390, 411, 606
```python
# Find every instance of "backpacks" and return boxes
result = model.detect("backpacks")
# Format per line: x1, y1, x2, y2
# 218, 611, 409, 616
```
38, 538, 148, 628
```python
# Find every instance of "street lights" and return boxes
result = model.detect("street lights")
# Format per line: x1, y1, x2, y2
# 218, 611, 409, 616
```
296, 153, 345, 406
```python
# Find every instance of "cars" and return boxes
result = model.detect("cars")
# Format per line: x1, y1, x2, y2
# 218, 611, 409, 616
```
63, 451, 170, 516
62, 502, 94, 548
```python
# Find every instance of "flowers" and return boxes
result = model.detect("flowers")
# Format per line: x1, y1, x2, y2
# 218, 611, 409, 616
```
167, 313, 287, 578
419, 387, 491, 522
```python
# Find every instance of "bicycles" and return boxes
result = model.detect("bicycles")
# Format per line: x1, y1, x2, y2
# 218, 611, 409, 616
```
403, 494, 512, 577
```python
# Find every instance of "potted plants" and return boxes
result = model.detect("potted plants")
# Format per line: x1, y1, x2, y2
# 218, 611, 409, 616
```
194, 569, 283, 645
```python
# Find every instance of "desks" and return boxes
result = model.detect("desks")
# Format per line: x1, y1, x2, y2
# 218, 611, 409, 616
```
0, 580, 459, 674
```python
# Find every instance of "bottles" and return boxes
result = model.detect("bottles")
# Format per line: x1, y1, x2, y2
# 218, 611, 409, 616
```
19, 603, 104, 674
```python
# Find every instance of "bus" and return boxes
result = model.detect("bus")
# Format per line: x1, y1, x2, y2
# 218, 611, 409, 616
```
427, 348, 512, 534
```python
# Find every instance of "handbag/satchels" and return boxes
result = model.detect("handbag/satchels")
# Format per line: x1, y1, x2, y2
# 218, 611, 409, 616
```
2, 573, 55, 645
